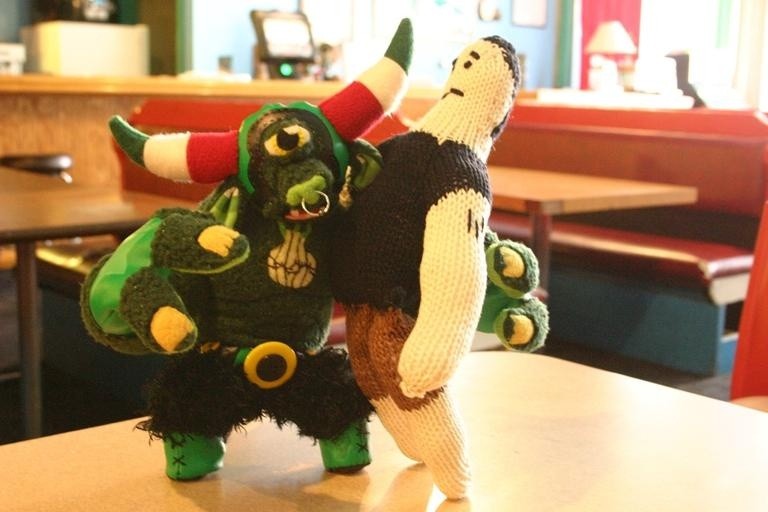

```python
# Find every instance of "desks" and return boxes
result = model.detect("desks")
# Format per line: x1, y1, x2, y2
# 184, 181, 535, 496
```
0, 164, 201, 438
487, 166, 699, 297
3, 350, 768, 511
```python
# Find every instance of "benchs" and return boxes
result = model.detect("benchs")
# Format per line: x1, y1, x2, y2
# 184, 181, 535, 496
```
487, 102, 768, 377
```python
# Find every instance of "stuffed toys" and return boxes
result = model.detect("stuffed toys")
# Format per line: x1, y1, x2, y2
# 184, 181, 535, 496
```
78, 18, 550, 482
330, 35, 523, 502
